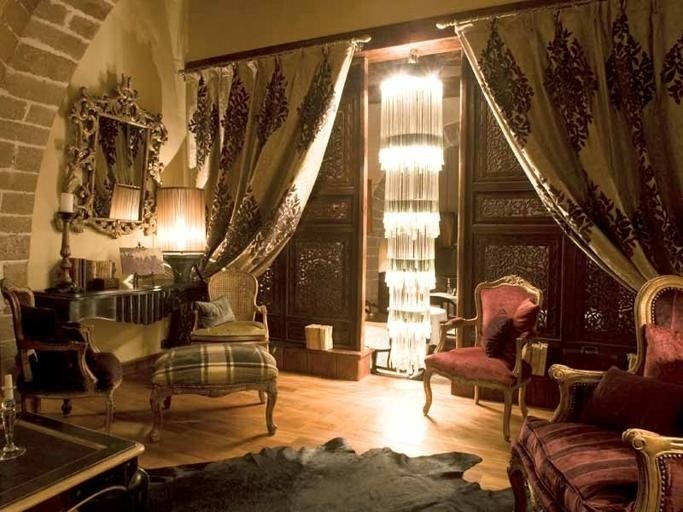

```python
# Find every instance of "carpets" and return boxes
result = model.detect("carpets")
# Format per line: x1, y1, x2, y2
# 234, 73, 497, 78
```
145, 436, 518, 511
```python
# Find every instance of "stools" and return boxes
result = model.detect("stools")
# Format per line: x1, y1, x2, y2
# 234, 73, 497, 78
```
144, 344, 280, 442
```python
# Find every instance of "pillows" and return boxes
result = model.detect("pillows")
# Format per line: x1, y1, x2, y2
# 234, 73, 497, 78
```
481, 299, 540, 361
578, 325, 683, 436
196, 294, 236, 327
69, 256, 116, 291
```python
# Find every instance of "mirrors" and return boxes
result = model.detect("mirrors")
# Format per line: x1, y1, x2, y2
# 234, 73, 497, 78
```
55, 74, 167, 240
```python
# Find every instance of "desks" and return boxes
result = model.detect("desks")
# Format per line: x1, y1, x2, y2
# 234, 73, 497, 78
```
35, 278, 178, 318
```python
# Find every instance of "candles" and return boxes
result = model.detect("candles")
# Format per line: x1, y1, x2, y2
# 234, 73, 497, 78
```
59, 191, 75, 212
4, 374, 16, 402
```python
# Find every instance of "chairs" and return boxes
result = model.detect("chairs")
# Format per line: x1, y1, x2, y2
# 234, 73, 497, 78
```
417, 275, 543, 442
189, 269, 271, 353
499, 275, 683, 512
3, 277, 121, 434
364, 302, 392, 375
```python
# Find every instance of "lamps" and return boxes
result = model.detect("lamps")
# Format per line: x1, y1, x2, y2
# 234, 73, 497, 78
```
109, 182, 140, 219
379, 74, 443, 378
155, 184, 210, 252
